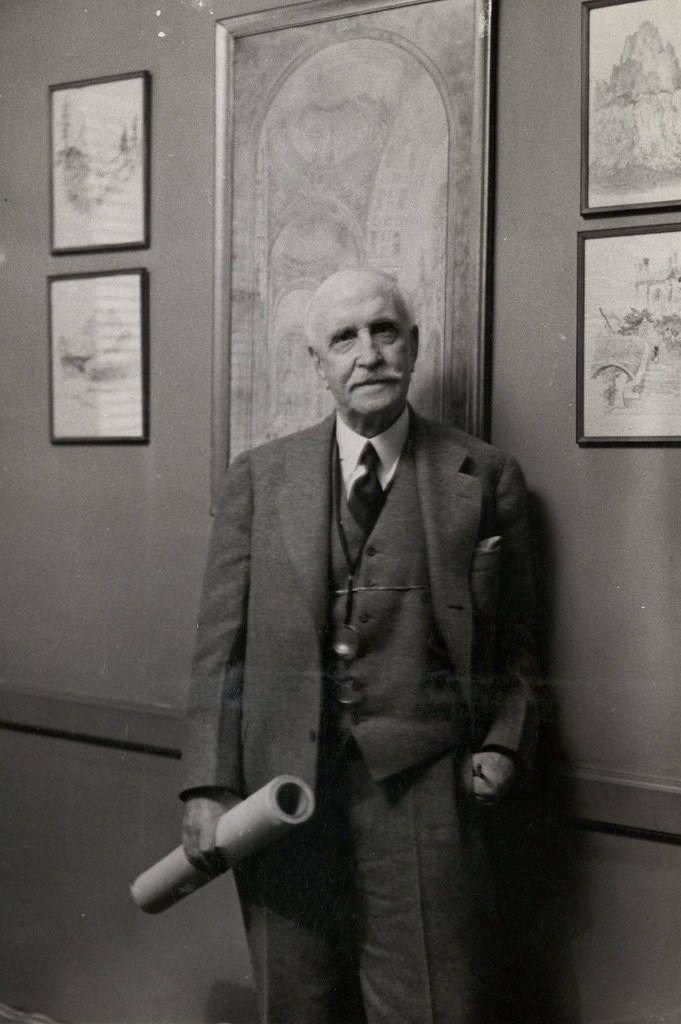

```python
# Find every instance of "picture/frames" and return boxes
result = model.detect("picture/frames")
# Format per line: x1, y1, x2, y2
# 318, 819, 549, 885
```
45, 268, 149, 446
574, 222, 681, 447
209, 1, 496, 522
48, 71, 150, 256
580, 0, 681, 216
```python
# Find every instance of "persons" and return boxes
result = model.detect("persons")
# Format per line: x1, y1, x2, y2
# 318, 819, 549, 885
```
178, 273, 537, 1024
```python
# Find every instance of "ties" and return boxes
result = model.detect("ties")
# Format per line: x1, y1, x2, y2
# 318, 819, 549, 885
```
347, 442, 385, 530
